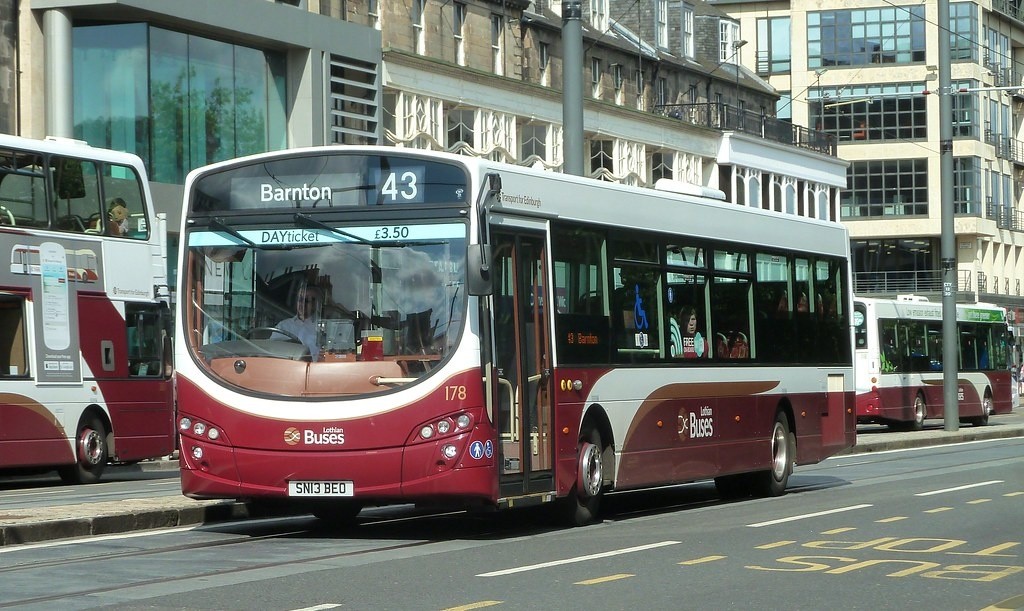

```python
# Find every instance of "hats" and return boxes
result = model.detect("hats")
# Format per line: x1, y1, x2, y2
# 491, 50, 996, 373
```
112, 205, 129, 220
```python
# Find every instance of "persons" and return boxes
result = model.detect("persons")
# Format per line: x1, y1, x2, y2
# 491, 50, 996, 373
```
614, 263, 650, 312
108, 198, 130, 235
268, 285, 326, 363
676, 304, 709, 357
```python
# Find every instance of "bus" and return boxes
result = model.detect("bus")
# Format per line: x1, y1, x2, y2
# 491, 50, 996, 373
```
0, 134, 176, 486
853, 293, 1015, 432
174, 143, 857, 531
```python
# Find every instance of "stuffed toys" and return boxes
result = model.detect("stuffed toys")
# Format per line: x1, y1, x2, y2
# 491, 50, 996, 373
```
112, 205, 129, 233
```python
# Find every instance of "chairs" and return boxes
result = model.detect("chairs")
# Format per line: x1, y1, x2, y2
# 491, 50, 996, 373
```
89, 211, 115, 236
769, 289, 824, 320
578, 290, 605, 316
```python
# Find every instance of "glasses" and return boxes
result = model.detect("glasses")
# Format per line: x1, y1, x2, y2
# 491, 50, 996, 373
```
298, 296, 315, 301
